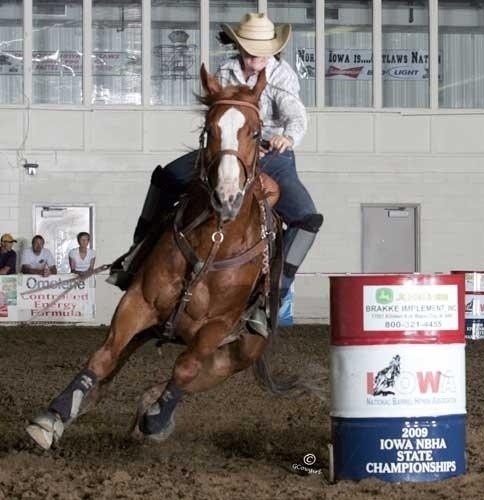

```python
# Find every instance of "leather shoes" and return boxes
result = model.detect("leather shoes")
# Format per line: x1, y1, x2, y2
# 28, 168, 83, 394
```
247, 306, 271, 339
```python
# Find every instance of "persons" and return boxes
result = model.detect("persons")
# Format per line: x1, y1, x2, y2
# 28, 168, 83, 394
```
68, 231, 96, 272
105, 11, 324, 340
0, 234, 18, 275
20, 235, 57, 276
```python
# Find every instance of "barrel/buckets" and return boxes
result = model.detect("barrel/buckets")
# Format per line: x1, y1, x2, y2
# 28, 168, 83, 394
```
450, 270, 484, 341
328, 272, 468, 485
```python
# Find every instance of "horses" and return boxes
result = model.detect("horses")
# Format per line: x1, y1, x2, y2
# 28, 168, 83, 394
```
26, 62, 332, 451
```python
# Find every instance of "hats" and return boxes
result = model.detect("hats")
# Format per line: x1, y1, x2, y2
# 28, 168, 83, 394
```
219, 11, 292, 59
1, 233, 18, 244
